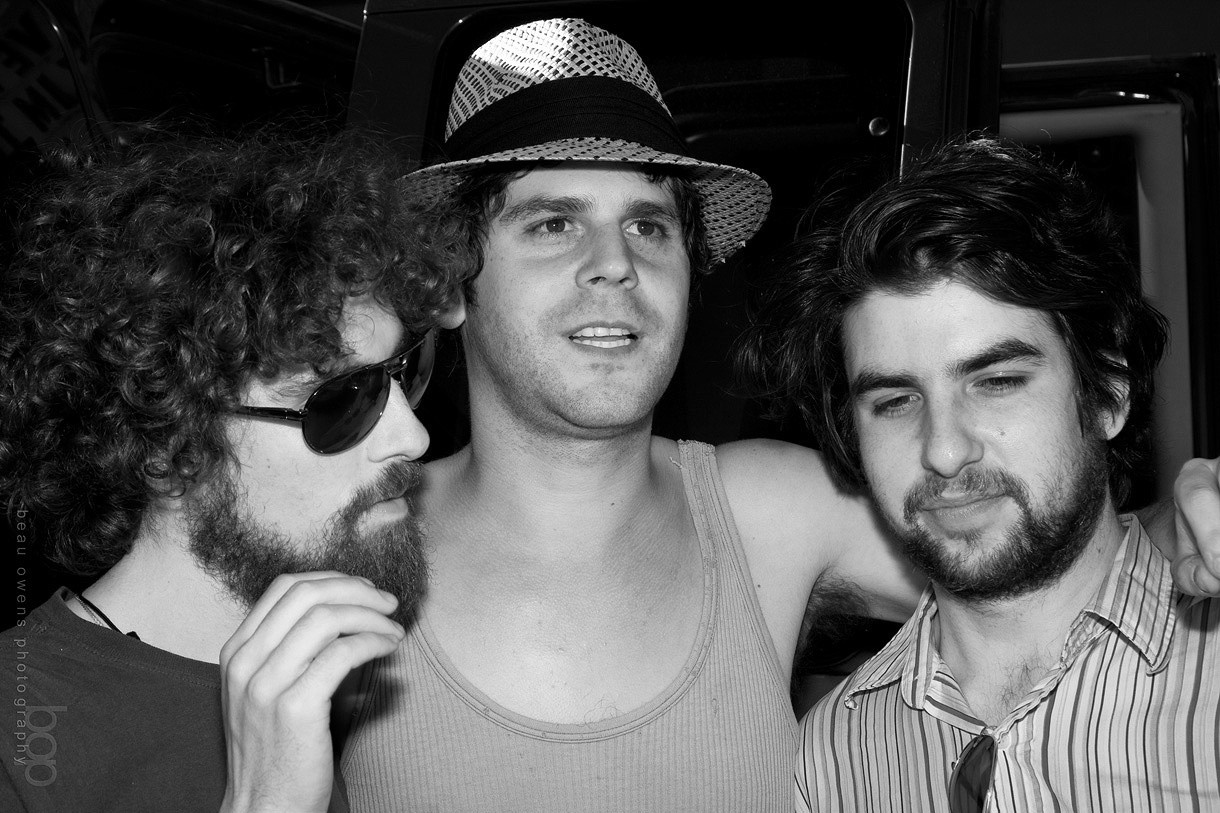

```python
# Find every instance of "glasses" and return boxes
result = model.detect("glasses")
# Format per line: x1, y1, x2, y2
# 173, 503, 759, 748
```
208, 326, 437, 456
943, 724, 1001, 812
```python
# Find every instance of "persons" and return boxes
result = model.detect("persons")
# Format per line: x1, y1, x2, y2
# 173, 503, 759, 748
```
790, 193, 1220, 812
4, 149, 481, 810
339, 14, 1220, 812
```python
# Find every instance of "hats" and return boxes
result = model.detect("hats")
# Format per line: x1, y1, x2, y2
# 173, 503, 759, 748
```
393, 14, 767, 270
